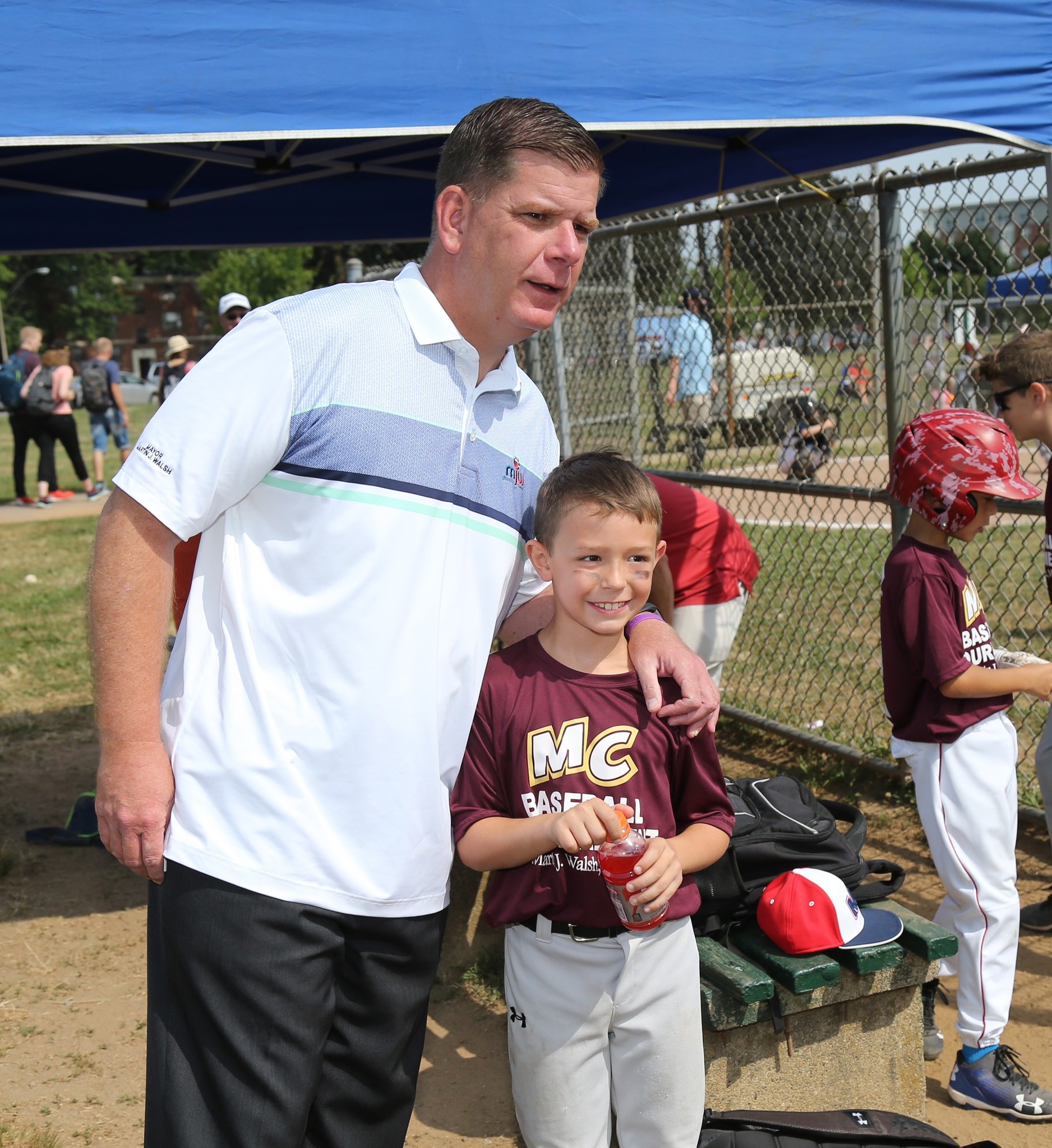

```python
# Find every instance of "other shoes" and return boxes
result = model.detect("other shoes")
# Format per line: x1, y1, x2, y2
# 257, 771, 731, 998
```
87, 482, 108, 501
16, 495, 35, 506
46, 488, 75, 503
36, 498, 55, 508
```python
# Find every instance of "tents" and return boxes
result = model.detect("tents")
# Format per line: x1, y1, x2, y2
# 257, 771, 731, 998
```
1, 1, 1052, 305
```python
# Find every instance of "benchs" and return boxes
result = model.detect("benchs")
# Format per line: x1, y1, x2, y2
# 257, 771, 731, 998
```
698, 898, 959, 1126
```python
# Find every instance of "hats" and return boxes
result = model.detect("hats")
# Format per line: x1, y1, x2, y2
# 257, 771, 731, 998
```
164, 334, 195, 359
682, 285, 711, 301
790, 396, 820, 419
217, 292, 252, 317
756, 867, 904, 955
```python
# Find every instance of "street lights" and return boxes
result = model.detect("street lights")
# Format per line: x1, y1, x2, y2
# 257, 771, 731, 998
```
0, 267, 49, 363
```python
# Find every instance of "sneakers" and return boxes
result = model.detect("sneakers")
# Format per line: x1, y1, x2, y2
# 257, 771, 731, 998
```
922, 977, 951, 1063
946, 1044, 1052, 1125
1020, 893, 1052, 932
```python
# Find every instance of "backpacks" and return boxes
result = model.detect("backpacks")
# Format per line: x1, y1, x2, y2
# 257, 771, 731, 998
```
24, 363, 63, 418
0, 353, 34, 414
690, 774, 907, 936
698, 1108, 1003, 1148
158, 358, 190, 406
81, 360, 115, 414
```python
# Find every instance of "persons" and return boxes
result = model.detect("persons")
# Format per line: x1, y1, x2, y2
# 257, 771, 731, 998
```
86, 97, 723, 1148
7, 293, 252, 506
646, 473, 761, 689
981, 326, 1051, 931
736, 321, 981, 486
663, 285, 719, 489
447, 449, 736, 1148
881, 408, 1052, 1121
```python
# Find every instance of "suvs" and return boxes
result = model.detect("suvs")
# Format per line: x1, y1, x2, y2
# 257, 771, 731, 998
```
146, 362, 175, 385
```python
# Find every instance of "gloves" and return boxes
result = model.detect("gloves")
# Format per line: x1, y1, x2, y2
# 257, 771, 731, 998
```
993, 645, 1051, 669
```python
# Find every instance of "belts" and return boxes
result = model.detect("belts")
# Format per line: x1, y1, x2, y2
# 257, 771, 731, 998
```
520, 912, 631, 943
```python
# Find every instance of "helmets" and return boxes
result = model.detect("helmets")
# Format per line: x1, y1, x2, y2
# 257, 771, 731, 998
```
886, 407, 1044, 538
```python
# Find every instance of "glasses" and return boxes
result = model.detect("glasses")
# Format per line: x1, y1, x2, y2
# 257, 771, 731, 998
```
993, 378, 1052, 411
224, 313, 246, 320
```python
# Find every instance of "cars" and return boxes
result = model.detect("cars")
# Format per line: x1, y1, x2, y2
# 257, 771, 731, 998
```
118, 371, 160, 404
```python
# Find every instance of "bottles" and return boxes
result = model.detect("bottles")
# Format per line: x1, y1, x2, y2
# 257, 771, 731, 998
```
599, 809, 669, 933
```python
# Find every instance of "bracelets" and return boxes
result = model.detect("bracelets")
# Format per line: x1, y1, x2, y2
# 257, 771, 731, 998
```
624, 613, 667, 638
